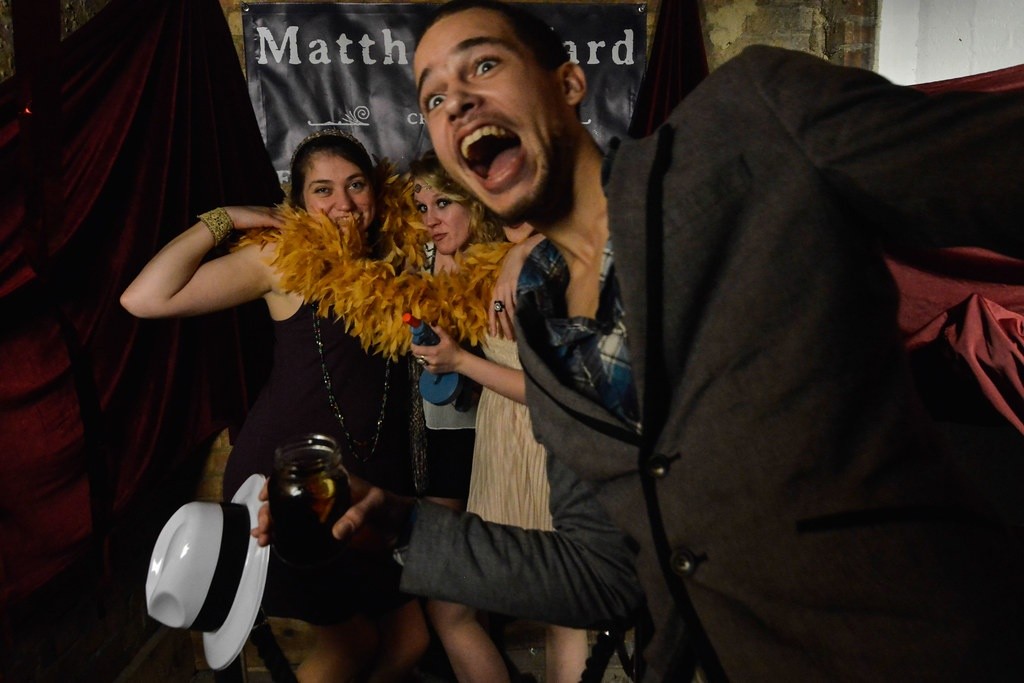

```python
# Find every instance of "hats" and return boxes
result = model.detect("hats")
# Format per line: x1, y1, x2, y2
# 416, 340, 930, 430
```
144, 473, 271, 669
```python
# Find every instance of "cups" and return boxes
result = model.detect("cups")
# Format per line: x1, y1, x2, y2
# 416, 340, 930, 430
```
268, 435, 350, 569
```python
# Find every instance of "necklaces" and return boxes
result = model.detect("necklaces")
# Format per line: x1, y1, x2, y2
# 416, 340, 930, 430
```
313, 301, 391, 461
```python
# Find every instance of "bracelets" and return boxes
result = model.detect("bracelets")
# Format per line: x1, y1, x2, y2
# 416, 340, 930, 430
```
195, 208, 236, 246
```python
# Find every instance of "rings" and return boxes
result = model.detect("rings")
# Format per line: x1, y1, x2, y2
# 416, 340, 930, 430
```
494, 301, 505, 311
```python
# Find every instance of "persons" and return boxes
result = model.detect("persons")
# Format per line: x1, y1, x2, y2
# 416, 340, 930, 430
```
429, 222, 587, 683
251, 1, 1022, 683
121, 122, 411, 680
401, 148, 490, 510
